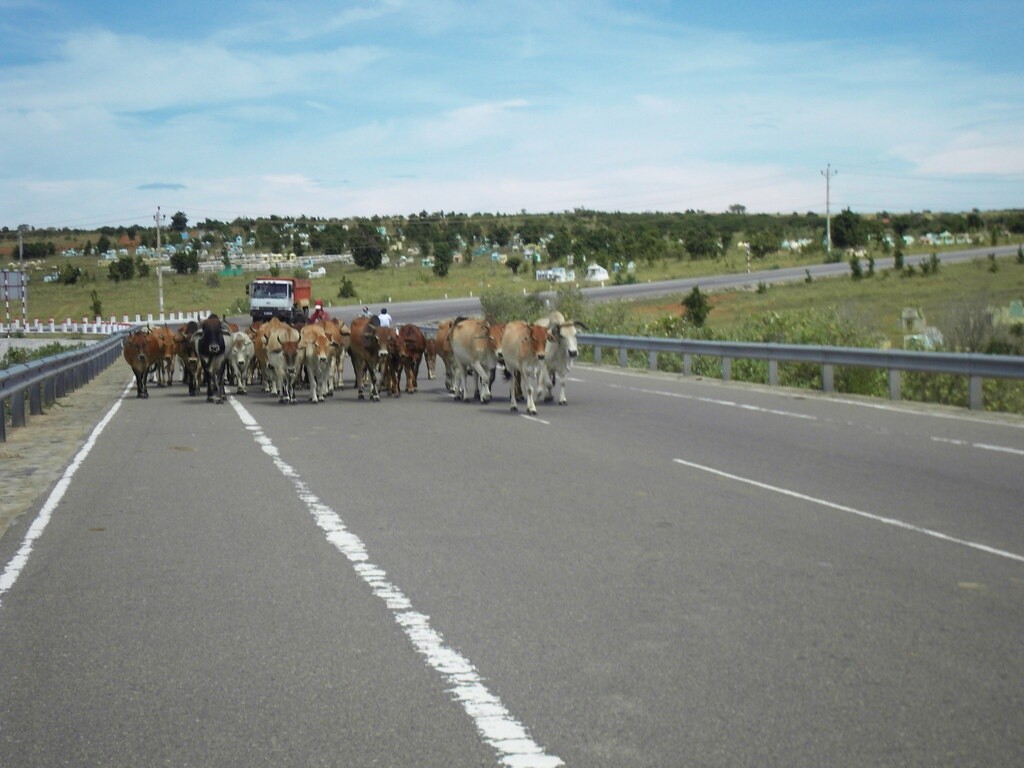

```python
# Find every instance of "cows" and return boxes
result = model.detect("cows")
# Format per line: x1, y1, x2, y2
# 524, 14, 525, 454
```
121, 311, 588, 416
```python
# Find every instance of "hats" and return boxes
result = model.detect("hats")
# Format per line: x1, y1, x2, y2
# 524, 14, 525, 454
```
315, 305, 321, 310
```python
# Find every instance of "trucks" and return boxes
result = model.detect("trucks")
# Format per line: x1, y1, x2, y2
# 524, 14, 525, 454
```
245, 275, 313, 324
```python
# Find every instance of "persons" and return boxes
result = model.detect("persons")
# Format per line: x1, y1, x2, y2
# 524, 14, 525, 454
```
309, 305, 329, 324
377, 308, 391, 328
359, 307, 372, 318
